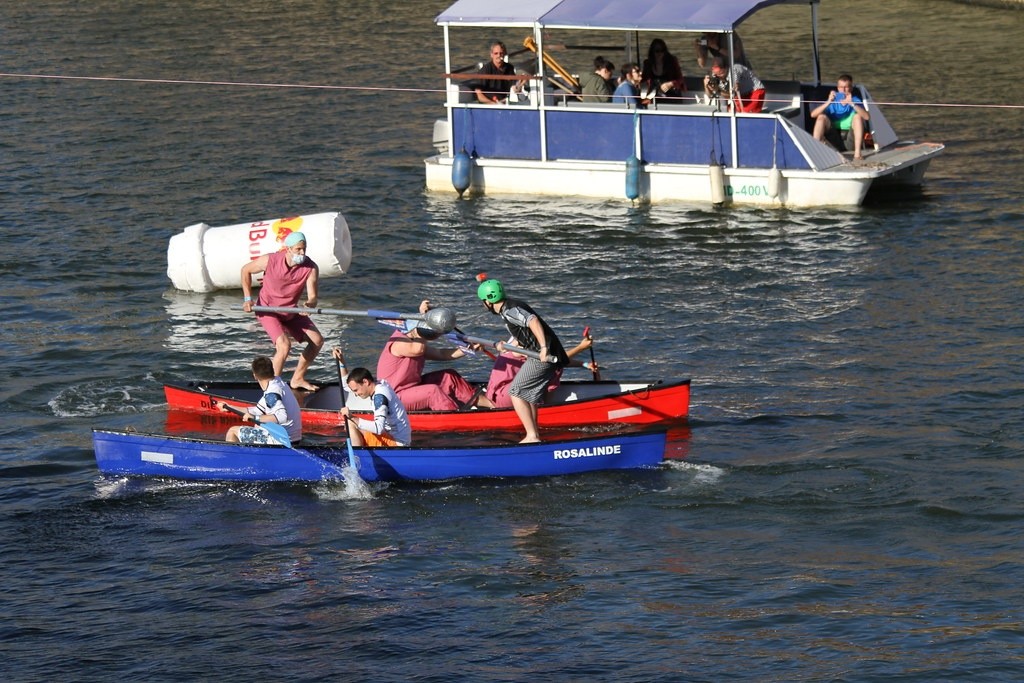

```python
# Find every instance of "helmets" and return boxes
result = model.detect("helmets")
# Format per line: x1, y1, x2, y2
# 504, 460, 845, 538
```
477, 279, 505, 304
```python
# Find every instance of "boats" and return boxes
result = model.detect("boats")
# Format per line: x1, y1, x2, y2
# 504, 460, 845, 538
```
91, 424, 670, 480
423, 0, 946, 206
161, 376, 691, 428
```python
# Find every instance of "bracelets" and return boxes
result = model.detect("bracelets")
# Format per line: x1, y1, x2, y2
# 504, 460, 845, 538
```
244, 297, 253, 301
254, 415, 260, 421
581, 360, 589, 368
717, 46, 721, 52
540, 345, 548, 349
852, 104, 856, 108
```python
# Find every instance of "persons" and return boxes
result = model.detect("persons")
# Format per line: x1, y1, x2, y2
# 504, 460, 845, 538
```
240, 231, 326, 393
470, 41, 516, 105
579, 55, 615, 104
704, 57, 765, 113
475, 278, 570, 444
216, 355, 303, 446
376, 300, 496, 414
810, 75, 870, 163
612, 63, 642, 104
331, 346, 412, 448
485, 335, 601, 408
641, 38, 687, 104
695, 31, 752, 71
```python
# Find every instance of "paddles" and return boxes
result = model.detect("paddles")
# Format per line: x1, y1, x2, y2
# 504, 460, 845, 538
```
209, 393, 292, 449
582, 325, 602, 380
522, 36, 584, 102
425, 304, 496, 362
335, 354, 362, 484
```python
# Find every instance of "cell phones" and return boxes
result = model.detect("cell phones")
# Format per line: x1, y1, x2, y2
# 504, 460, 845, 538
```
835, 93, 846, 99
698, 39, 707, 45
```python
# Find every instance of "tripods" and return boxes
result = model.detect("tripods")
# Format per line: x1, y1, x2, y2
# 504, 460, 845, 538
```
710, 93, 720, 112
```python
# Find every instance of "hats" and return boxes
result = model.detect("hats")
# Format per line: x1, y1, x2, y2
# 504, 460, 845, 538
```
711, 56, 730, 67
284, 232, 307, 247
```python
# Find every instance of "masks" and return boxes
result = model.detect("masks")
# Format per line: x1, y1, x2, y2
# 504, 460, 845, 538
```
288, 248, 307, 264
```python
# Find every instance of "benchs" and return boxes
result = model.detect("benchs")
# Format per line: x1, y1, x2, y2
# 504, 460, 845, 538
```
451, 76, 805, 130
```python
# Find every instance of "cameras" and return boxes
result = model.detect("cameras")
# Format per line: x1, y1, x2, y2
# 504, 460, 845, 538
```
708, 77, 719, 86
